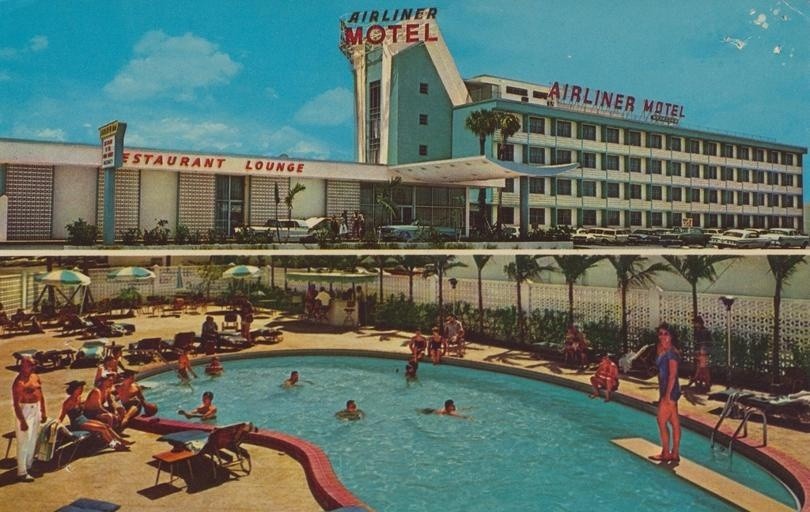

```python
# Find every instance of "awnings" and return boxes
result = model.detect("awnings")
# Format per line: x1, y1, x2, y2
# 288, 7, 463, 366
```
285, 271, 379, 284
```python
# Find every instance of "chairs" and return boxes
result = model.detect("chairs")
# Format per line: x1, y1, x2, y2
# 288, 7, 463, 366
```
442, 330, 466, 357
54, 498, 121, 512
39, 416, 102, 472
156, 421, 252, 482
610, 343, 657, 380
706, 389, 810, 429
0, 292, 283, 374
532, 341, 595, 366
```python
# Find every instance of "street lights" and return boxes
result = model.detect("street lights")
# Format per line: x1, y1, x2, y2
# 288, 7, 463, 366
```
448, 275, 462, 321
717, 290, 738, 405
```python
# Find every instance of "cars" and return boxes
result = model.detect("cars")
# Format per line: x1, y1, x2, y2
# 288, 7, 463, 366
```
569, 220, 807, 252
375, 216, 463, 244
234, 215, 336, 241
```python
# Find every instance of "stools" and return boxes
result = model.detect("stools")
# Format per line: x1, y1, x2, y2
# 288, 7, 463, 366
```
343, 308, 356, 331
152, 449, 195, 489
2, 431, 17, 461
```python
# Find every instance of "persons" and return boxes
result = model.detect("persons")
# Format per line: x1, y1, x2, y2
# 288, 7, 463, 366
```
405, 313, 464, 383
240, 223, 254, 237
11, 297, 365, 484
331, 209, 364, 241
562, 315, 711, 460
304, 284, 367, 327
143, 226, 215, 245
0, 302, 128, 339
437, 399, 459, 416
